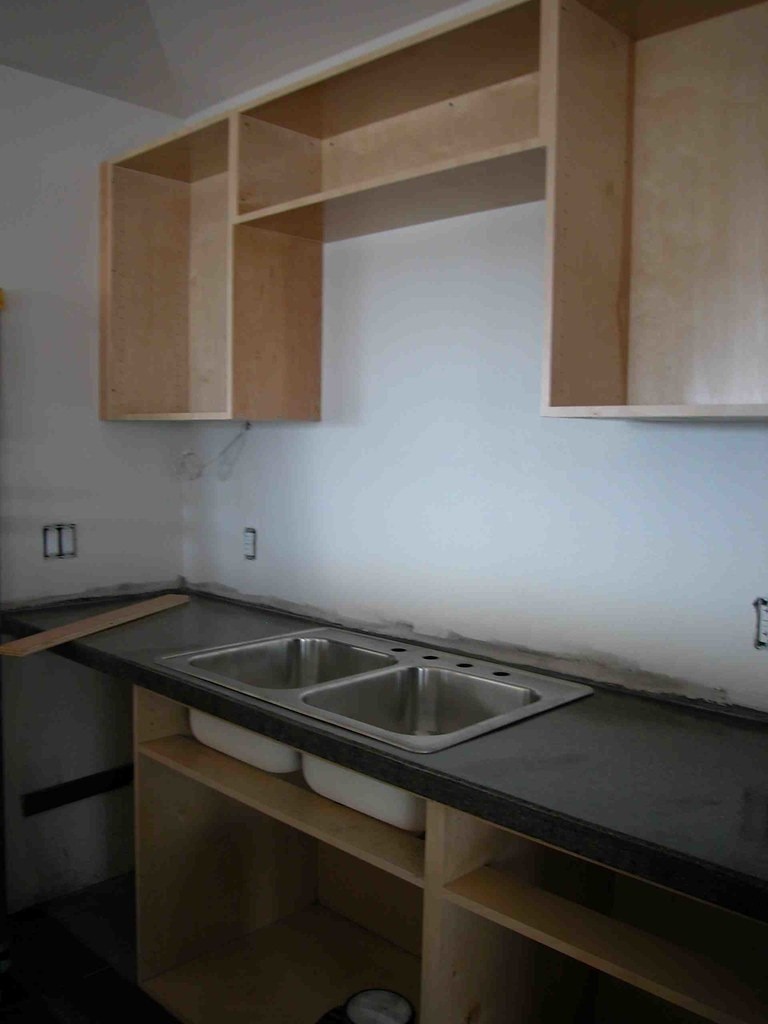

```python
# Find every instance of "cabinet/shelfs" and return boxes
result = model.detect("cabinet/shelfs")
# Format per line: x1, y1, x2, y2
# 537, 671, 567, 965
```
233, 0, 545, 243
541, 1, 767, 423
136, 677, 767, 1024
98, 110, 325, 422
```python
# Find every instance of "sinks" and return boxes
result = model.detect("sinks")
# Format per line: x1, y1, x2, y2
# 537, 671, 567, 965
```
300, 665, 597, 835
166, 635, 399, 772
